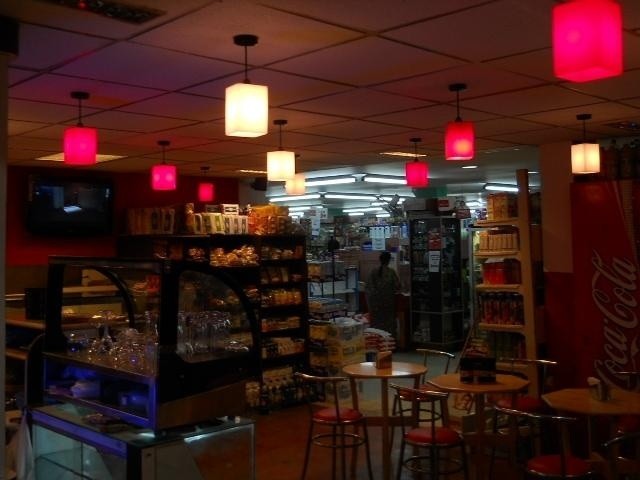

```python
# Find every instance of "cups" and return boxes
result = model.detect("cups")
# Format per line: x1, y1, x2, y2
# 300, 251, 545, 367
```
66, 331, 89, 359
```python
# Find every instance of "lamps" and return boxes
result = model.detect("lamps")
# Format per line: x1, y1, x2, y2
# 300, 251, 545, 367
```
223, 34, 269, 141
569, 114, 603, 178
150, 139, 177, 192
196, 166, 216, 202
286, 154, 306, 195
267, 118, 294, 182
62, 91, 101, 168
549, 0, 626, 81
404, 137, 430, 189
444, 83, 474, 160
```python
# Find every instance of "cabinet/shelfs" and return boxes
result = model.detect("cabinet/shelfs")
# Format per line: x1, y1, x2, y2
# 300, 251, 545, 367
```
4, 184, 306, 433
28, 402, 257, 480
307, 189, 469, 344
468, 188, 539, 409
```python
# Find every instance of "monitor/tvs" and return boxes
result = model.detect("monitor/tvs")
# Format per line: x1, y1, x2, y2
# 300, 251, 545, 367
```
22, 168, 115, 239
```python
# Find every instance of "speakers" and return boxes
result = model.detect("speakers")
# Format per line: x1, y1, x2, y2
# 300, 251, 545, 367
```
254, 176, 267, 191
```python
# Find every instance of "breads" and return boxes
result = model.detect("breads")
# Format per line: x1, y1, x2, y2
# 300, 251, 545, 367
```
210, 245, 259, 267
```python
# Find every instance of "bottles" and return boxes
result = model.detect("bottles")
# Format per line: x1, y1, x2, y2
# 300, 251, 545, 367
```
177, 310, 231, 355
143, 308, 158, 373
261, 376, 297, 405
80, 275, 92, 297
422, 328, 430, 342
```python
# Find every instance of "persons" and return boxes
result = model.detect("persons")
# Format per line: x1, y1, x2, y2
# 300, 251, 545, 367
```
365, 253, 401, 353
328, 236, 340, 252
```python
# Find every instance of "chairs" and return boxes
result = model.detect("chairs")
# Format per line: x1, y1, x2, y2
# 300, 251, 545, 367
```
434, 344, 640, 479
391, 382, 468, 478
393, 348, 455, 436
294, 370, 375, 479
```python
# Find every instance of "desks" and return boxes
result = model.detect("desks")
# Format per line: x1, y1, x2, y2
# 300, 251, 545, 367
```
344, 361, 428, 478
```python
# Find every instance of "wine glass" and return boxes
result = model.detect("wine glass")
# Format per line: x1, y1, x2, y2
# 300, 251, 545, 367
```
89, 310, 118, 366
